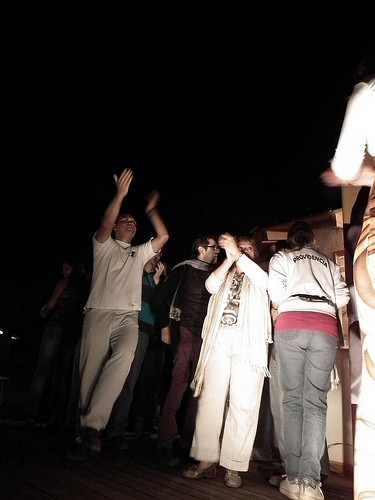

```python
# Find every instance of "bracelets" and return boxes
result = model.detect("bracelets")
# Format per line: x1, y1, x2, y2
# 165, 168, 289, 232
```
146, 205, 157, 217
235, 253, 243, 261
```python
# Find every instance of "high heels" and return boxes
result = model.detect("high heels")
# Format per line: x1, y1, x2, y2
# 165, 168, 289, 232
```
182, 463, 216, 478
224, 469, 241, 488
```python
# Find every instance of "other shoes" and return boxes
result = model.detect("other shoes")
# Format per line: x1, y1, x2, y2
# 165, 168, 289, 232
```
47, 417, 63, 425
249, 447, 272, 461
268, 473, 288, 487
25, 416, 34, 424
67, 426, 102, 460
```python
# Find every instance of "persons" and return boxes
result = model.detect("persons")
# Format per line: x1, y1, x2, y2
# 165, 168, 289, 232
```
320, 65, 375, 500
105, 221, 351, 500
73, 166, 170, 456
29, 258, 74, 424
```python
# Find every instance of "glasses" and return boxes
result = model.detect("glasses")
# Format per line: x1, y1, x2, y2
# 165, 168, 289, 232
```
202, 244, 218, 250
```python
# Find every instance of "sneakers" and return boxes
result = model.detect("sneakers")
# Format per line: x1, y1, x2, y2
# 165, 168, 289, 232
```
298, 475, 325, 500
279, 476, 300, 499
